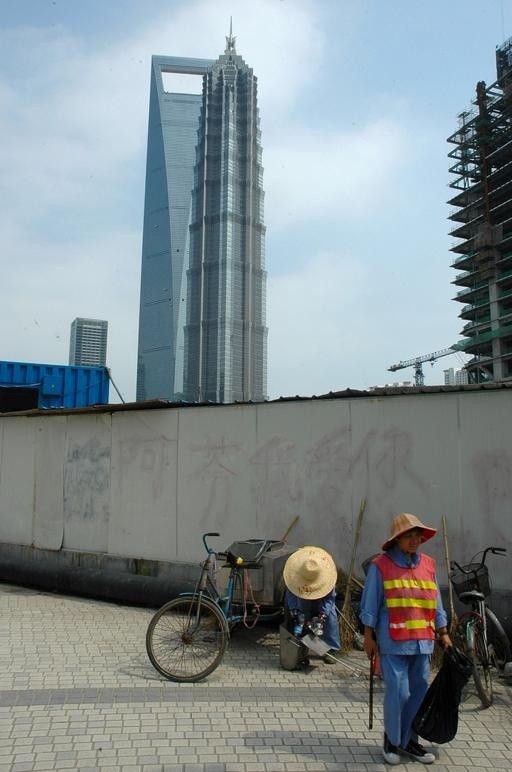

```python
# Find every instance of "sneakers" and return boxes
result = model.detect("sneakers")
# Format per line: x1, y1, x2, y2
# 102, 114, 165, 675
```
382, 732, 401, 765
399, 738, 435, 764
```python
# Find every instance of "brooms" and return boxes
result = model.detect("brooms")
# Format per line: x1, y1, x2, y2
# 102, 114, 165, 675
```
441, 515, 470, 661
338, 498, 364, 648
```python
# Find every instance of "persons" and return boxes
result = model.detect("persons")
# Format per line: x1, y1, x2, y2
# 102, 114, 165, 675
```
283, 544, 340, 665
356, 513, 456, 764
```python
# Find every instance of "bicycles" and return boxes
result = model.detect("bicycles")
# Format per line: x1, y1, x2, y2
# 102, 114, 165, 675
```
447, 544, 512, 709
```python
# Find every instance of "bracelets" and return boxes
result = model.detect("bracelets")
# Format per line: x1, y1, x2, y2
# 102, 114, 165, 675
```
438, 630, 449, 636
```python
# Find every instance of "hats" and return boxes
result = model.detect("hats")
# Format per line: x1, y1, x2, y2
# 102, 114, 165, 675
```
283, 546, 337, 601
379, 512, 437, 551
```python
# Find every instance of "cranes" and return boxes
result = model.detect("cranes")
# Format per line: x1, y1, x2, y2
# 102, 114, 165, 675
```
386, 345, 460, 388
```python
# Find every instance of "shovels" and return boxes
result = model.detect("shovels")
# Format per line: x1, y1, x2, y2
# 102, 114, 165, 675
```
300, 634, 362, 677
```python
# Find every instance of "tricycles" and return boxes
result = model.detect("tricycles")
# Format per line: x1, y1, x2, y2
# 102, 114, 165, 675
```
144, 531, 300, 684
